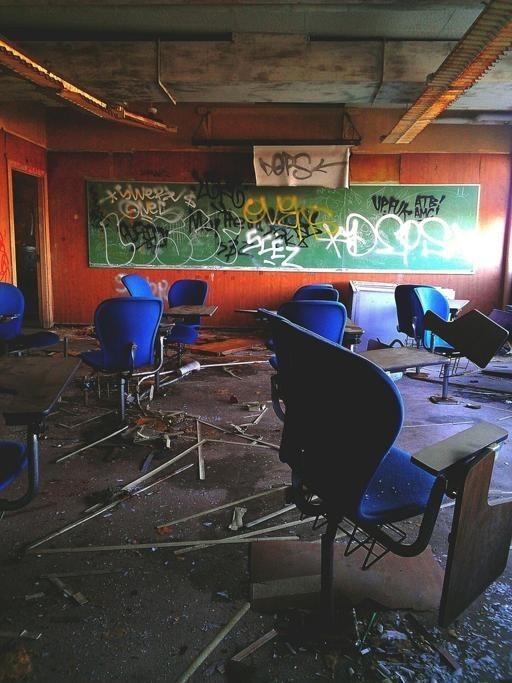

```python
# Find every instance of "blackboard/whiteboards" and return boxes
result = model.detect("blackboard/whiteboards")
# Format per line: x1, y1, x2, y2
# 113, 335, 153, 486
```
86, 180, 482, 275
